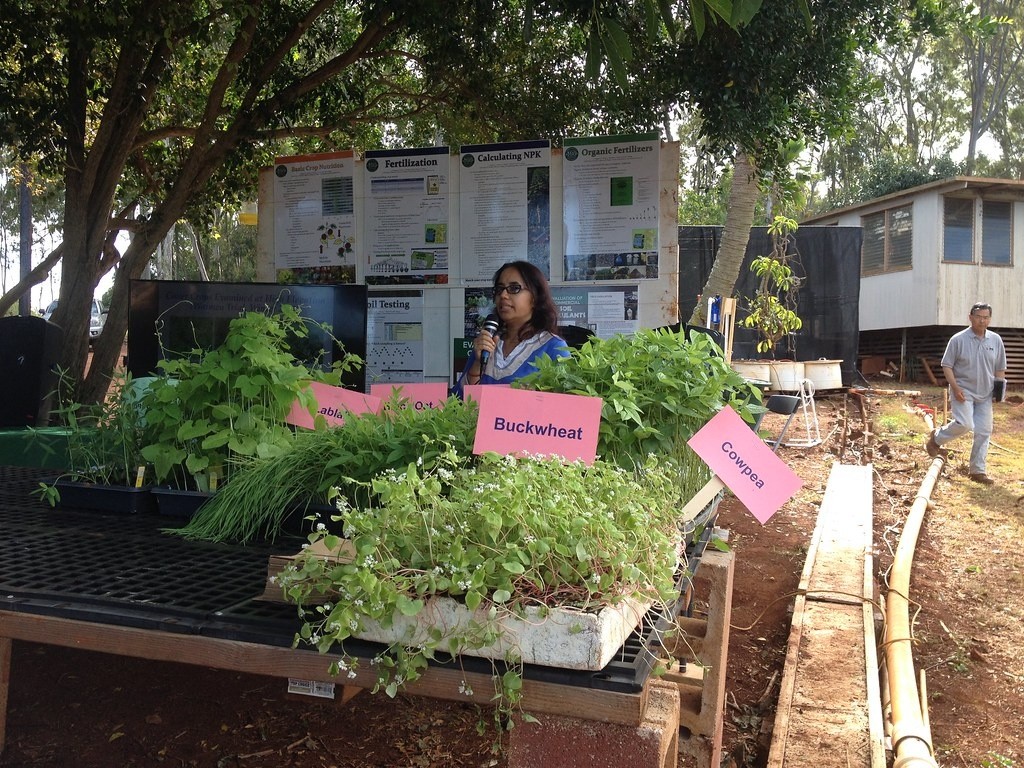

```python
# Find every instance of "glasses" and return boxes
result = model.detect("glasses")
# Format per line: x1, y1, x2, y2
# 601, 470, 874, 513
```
491, 284, 529, 294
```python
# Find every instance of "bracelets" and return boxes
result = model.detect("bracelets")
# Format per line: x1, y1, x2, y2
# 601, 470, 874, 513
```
468, 371, 482, 377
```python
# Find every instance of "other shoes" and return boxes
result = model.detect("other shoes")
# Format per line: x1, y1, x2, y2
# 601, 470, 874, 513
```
968, 473, 994, 484
926, 429, 940, 456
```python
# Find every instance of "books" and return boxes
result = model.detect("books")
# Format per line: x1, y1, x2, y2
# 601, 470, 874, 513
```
992, 377, 1007, 403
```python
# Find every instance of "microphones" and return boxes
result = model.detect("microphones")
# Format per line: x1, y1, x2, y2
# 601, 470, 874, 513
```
479, 314, 499, 379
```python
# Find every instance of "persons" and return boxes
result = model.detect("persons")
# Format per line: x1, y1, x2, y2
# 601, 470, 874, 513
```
926, 303, 1007, 485
450, 261, 574, 407
